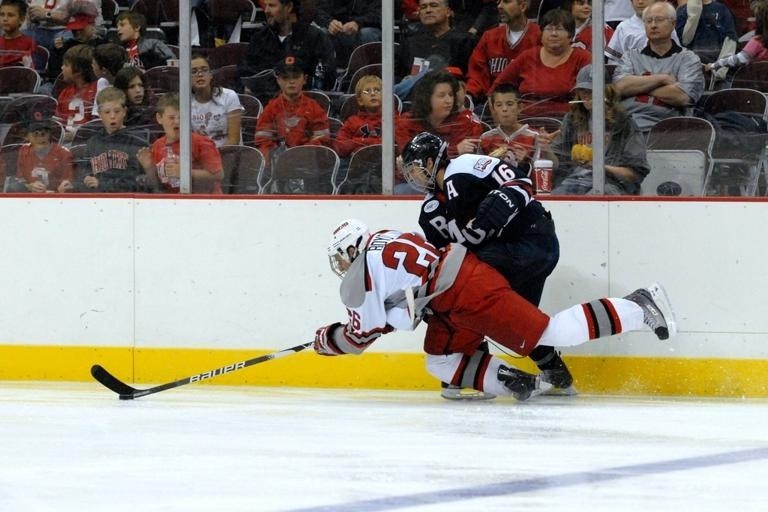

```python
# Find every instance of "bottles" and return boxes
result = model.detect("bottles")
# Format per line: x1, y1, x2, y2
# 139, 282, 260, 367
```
165, 143, 180, 189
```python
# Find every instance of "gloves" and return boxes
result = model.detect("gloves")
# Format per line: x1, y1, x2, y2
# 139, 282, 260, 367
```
313, 322, 346, 356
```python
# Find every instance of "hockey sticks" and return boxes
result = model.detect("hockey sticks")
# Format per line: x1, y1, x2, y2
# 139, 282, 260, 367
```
91, 339, 314, 400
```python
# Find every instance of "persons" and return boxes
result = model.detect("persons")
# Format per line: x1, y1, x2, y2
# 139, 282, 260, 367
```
314, 221, 678, 404
1, 0, 725, 198
397, 129, 560, 393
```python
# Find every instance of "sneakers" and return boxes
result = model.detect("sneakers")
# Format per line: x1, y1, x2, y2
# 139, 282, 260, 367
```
622, 289, 669, 339
537, 357, 574, 388
498, 365, 540, 401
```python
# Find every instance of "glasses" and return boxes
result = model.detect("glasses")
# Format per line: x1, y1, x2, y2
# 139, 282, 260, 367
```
643, 16, 673, 26
361, 87, 381, 96
190, 68, 210, 78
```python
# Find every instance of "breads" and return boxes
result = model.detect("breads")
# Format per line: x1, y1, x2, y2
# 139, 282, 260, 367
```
570, 144, 592, 166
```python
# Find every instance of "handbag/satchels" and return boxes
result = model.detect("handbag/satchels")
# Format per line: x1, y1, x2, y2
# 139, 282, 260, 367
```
700, 110, 768, 159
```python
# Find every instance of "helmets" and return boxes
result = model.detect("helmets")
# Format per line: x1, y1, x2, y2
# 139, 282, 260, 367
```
397, 132, 449, 193
326, 221, 372, 279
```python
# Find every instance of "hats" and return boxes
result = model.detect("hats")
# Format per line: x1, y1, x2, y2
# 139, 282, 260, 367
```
274, 50, 305, 75
64, 14, 95, 31
26, 109, 54, 133
569, 64, 610, 94
443, 66, 464, 76
